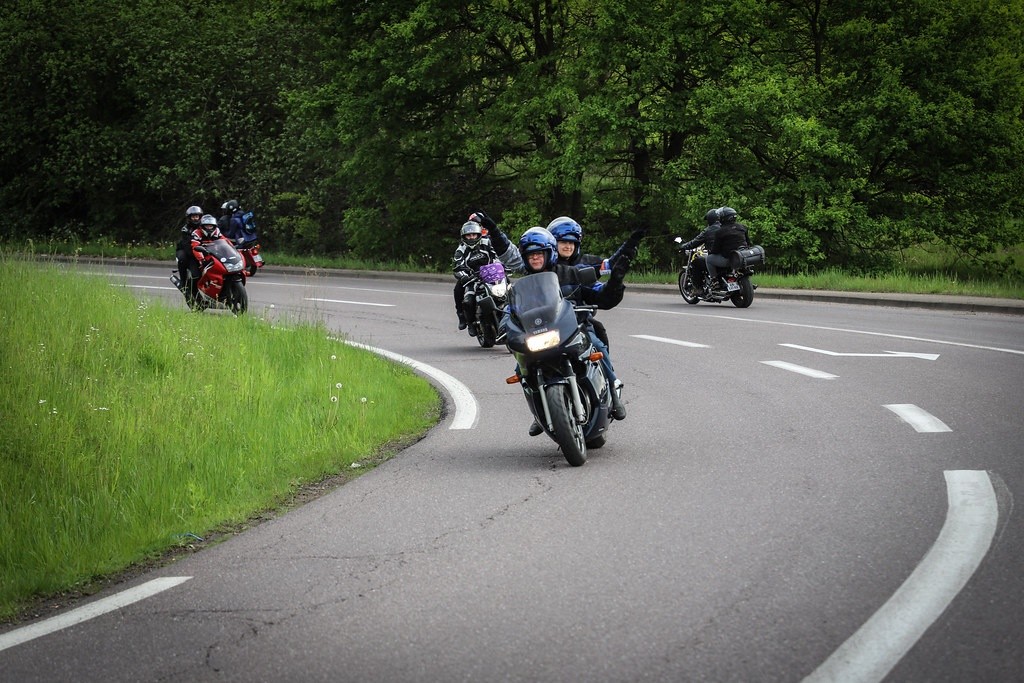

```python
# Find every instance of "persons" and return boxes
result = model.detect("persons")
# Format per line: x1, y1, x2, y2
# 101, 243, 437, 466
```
679, 209, 721, 293
475, 207, 640, 355
503, 226, 630, 436
188, 214, 238, 303
176, 205, 203, 289
452, 212, 509, 336
218, 200, 257, 248
705, 206, 751, 287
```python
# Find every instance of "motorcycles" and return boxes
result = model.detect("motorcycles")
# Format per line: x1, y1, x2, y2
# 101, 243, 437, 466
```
233, 244, 266, 277
451, 264, 512, 348
477, 271, 623, 463
169, 238, 249, 318
674, 236, 766, 308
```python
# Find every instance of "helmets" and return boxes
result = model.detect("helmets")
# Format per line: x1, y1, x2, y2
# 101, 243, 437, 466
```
518, 227, 558, 263
226, 200, 239, 213
469, 213, 489, 236
546, 217, 582, 261
704, 209, 719, 224
461, 222, 482, 248
201, 215, 218, 237
719, 207, 737, 222
186, 206, 203, 226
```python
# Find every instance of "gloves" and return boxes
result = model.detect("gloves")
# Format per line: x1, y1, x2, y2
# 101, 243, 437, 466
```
611, 255, 631, 280
475, 207, 496, 231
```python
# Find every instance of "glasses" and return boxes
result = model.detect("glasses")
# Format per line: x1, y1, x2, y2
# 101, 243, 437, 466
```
526, 251, 545, 259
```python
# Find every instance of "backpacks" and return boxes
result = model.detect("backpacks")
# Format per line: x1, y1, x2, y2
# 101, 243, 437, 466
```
232, 212, 257, 235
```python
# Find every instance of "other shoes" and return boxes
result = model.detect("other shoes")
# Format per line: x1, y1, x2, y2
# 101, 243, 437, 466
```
610, 388, 626, 420
689, 289, 703, 298
457, 312, 467, 329
710, 281, 719, 290
468, 323, 477, 337
529, 420, 546, 436
